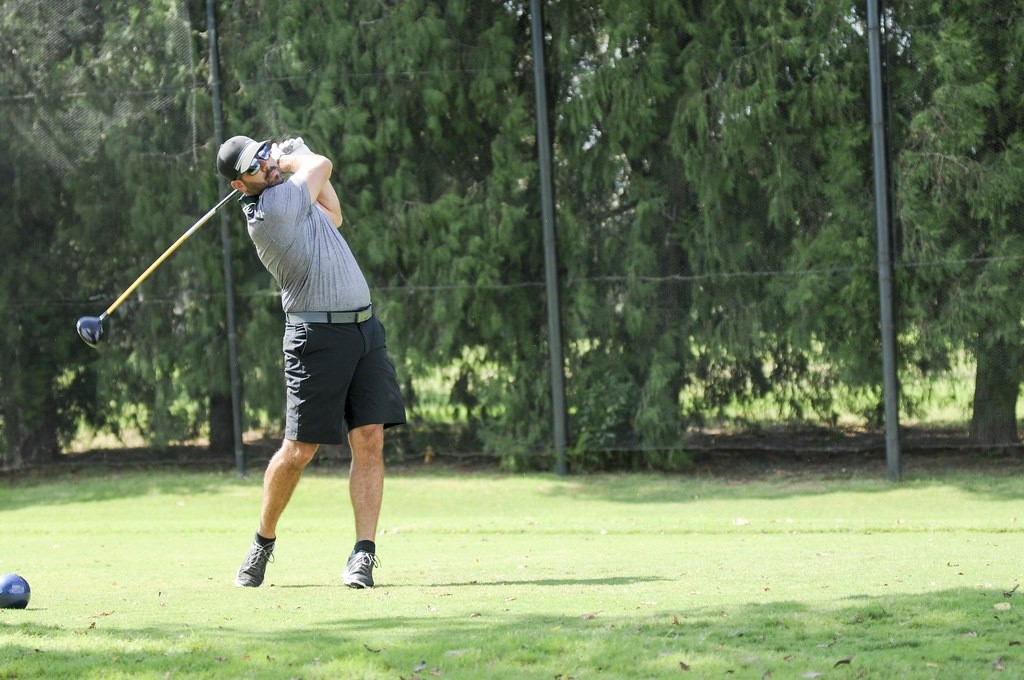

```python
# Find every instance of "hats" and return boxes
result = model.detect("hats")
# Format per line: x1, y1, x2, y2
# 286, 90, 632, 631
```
216, 133, 273, 180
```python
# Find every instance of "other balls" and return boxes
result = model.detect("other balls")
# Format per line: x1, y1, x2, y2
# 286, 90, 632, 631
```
0, 574, 31, 609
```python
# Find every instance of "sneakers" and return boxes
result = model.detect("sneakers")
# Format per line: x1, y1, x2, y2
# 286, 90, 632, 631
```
236, 539, 277, 589
340, 549, 381, 589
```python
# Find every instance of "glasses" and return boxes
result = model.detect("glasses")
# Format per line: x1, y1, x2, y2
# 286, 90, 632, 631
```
244, 145, 272, 177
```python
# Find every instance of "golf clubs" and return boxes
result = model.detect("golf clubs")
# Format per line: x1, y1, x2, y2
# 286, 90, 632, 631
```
75, 137, 304, 349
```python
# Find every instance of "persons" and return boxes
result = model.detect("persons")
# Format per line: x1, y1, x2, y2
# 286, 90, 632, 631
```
213, 134, 408, 591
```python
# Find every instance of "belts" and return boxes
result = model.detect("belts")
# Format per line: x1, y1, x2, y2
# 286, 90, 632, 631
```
287, 305, 374, 325
